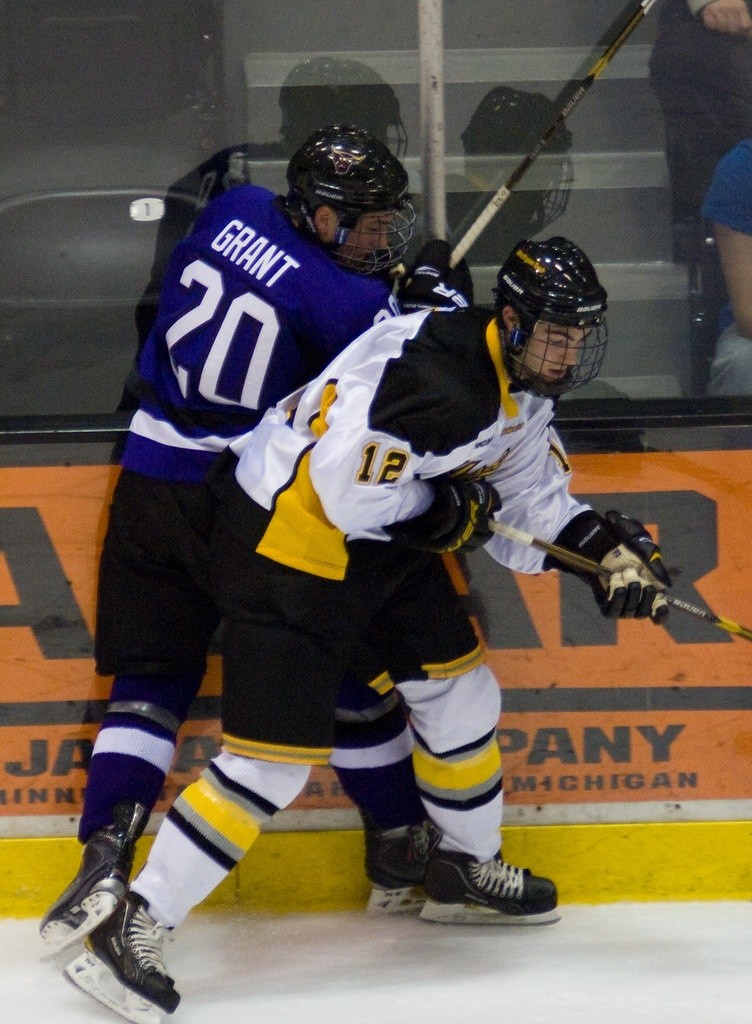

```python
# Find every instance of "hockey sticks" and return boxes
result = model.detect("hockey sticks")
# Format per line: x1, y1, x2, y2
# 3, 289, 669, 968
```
447, 2, 658, 269
486, 518, 751, 645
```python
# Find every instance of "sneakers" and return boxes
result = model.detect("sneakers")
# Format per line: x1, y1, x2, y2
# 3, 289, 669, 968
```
39, 800, 145, 950
419, 847, 562, 925
364, 819, 442, 916
62, 891, 180, 1024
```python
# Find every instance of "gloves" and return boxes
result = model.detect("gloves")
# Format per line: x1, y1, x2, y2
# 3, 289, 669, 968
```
544, 510, 674, 624
396, 239, 473, 314
382, 478, 502, 556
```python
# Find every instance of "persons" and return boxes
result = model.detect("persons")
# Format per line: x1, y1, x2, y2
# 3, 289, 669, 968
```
109, 55, 574, 463
650, 0, 752, 398
41, 128, 475, 956
61, 236, 671, 1024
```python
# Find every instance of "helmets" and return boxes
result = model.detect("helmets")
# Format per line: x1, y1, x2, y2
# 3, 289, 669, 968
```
497, 237, 608, 399
285, 125, 416, 276
460, 85, 575, 240
278, 57, 407, 165
